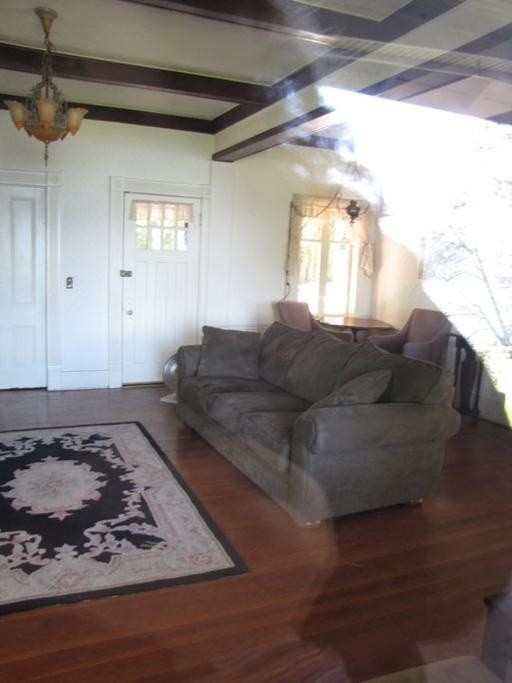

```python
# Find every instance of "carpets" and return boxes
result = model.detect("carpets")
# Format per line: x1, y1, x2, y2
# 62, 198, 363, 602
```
0, 420, 251, 616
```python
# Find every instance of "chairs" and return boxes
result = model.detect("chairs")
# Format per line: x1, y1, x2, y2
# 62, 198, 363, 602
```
278, 299, 454, 365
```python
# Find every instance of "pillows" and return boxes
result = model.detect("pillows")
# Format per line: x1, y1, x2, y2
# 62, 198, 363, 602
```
291, 368, 394, 426
194, 325, 262, 381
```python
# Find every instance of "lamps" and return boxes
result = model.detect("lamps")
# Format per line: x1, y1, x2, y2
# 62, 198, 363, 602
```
1, 5, 89, 167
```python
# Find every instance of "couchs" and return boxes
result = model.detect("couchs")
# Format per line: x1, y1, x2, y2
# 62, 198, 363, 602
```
175, 320, 463, 533
